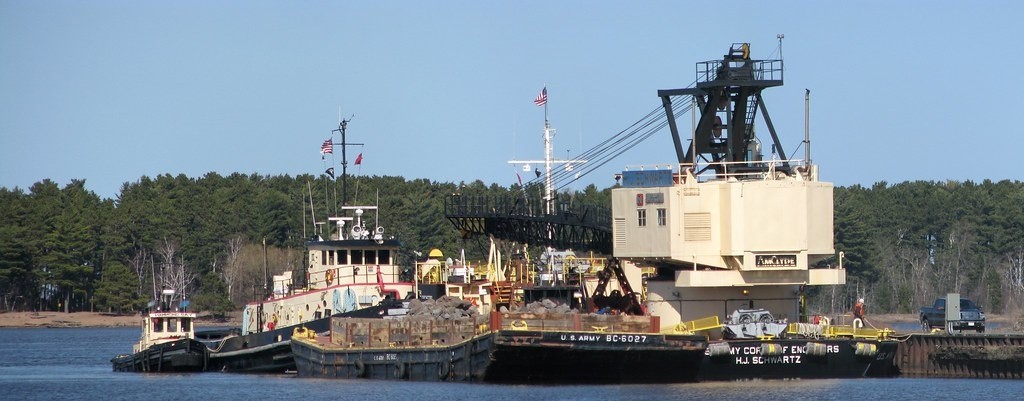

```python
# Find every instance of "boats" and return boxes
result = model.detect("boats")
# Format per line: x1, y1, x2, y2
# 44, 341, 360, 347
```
240, 113, 417, 335
132, 288, 196, 354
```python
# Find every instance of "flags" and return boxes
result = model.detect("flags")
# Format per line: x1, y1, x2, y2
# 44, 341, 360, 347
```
534, 86, 547, 107
320, 137, 333, 159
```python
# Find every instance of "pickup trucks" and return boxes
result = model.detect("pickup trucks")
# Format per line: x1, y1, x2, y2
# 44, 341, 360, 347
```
919, 298, 985, 333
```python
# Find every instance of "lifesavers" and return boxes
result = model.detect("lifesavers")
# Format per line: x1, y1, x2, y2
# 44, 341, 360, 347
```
438, 359, 449, 380
511, 319, 528, 330
325, 269, 334, 284
354, 359, 365, 378
394, 361, 405, 379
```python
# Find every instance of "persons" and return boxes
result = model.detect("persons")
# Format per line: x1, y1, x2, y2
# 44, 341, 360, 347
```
853, 299, 866, 327
